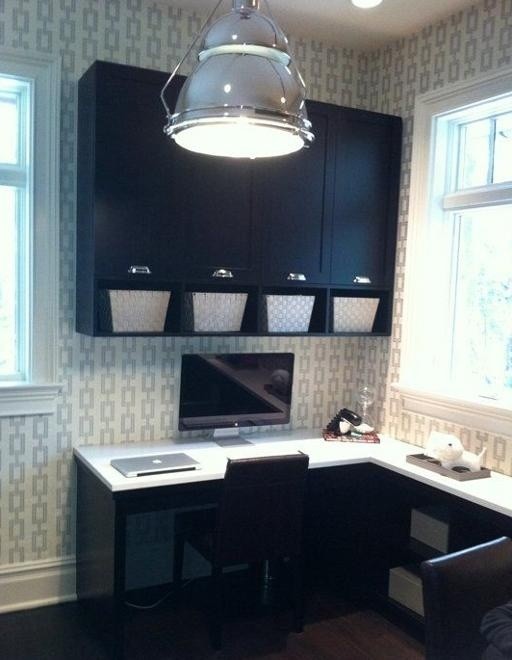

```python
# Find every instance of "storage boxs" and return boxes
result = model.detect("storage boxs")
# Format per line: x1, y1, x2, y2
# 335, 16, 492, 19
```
407, 502, 468, 555
388, 563, 424, 617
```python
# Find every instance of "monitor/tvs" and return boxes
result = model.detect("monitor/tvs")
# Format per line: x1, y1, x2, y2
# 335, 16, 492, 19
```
179, 353, 295, 448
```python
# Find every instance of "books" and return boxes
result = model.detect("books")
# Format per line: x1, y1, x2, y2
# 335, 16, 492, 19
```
322, 428, 380, 443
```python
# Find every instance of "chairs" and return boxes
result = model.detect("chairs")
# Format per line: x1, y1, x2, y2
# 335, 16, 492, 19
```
420, 535, 511, 660
173, 453, 310, 651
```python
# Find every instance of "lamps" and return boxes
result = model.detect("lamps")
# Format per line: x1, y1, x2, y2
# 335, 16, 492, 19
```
160, 0, 316, 161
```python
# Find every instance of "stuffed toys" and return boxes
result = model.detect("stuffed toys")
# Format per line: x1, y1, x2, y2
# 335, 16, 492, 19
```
424, 430, 487, 472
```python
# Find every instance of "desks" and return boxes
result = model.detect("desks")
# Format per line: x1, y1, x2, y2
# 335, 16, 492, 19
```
77, 430, 512, 659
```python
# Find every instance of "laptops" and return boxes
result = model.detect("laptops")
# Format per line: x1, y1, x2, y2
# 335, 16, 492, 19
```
110, 452, 202, 478
